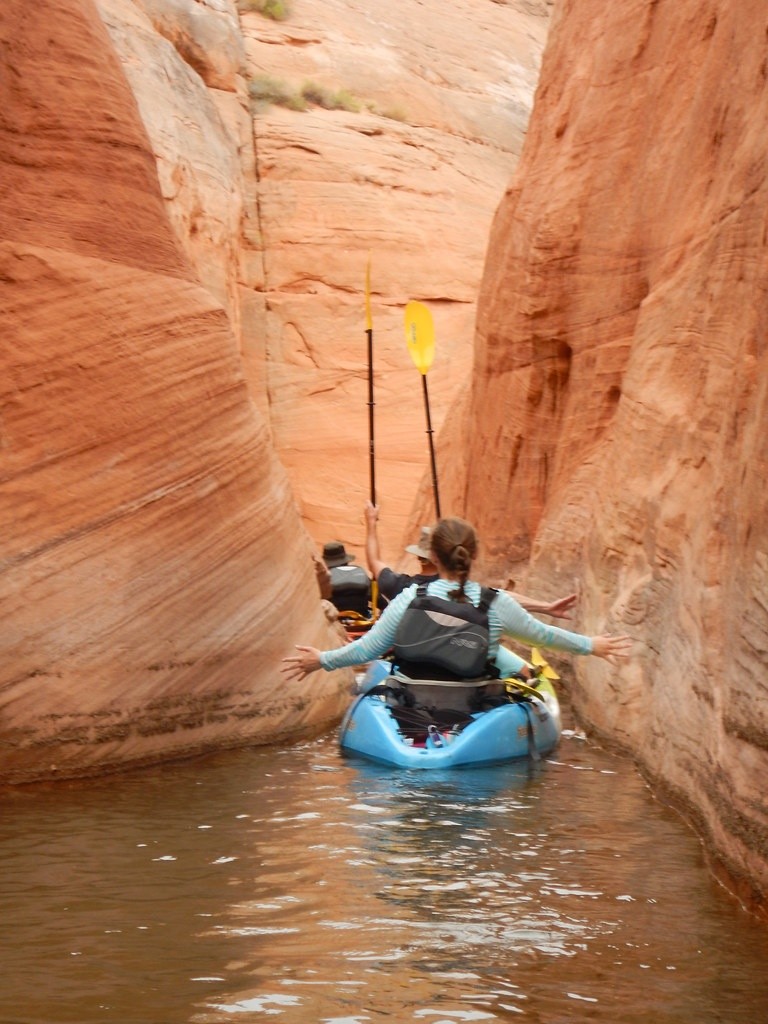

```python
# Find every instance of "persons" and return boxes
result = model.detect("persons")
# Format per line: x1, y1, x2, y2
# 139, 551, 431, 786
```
321, 541, 388, 631
280, 516, 632, 682
364, 500, 578, 620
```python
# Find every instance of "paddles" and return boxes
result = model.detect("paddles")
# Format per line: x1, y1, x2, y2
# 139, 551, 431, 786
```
364, 250, 378, 631
504, 645, 563, 705
400, 302, 442, 524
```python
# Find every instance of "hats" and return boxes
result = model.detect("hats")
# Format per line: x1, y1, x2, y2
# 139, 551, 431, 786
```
321, 542, 356, 567
403, 527, 434, 559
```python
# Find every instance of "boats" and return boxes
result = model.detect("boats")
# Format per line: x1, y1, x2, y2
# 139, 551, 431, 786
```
338, 645, 561, 769
